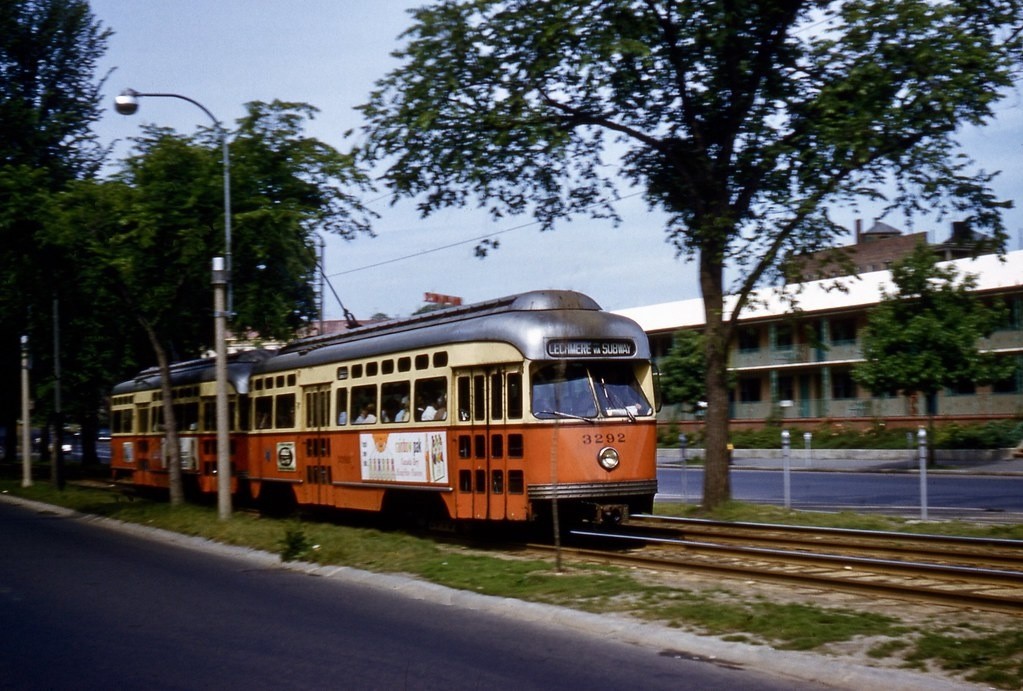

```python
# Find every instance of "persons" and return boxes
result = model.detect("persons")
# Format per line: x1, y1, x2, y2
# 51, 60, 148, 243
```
337, 390, 447, 424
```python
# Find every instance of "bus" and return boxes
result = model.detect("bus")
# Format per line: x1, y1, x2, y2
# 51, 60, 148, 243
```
109, 290, 659, 547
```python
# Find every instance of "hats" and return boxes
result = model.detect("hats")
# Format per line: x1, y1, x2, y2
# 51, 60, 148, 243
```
402, 396, 409, 404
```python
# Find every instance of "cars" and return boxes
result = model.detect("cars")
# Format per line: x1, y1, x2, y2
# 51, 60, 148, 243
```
30, 428, 75, 455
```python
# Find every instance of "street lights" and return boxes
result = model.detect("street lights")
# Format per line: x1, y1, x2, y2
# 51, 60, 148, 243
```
114, 88, 231, 521
